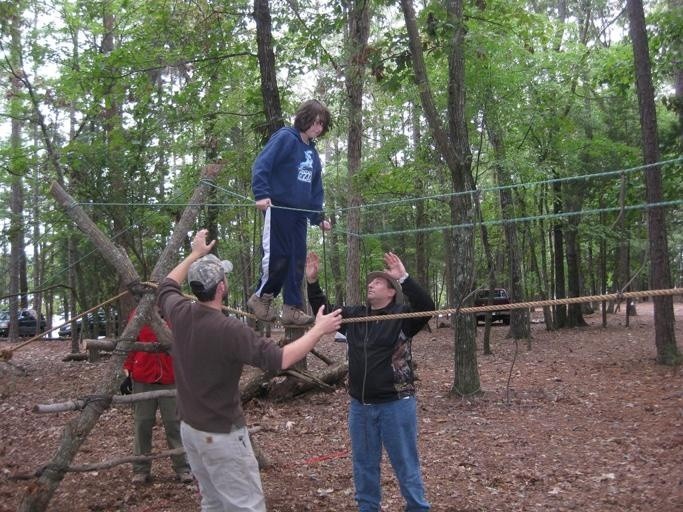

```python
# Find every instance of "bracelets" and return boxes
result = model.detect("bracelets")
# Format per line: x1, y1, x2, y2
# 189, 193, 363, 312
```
397, 273, 409, 284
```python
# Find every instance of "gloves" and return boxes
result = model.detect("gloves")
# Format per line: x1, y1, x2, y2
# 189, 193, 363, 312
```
119, 372, 134, 397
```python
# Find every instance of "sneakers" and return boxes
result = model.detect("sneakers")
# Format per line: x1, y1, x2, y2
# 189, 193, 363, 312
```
280, 304, 314, 326
246, 292, 277, 323
131, 473, 152, 484
175, 470, 193, 483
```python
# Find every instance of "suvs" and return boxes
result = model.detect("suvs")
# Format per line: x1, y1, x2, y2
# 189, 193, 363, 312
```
471, 289, 511, 325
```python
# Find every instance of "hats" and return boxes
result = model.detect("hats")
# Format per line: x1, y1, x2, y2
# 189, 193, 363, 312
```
187, 254, 233, 290
366, 269, 401, 299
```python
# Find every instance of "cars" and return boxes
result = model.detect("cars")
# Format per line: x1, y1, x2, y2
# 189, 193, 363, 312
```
59, 312, 117, 338
0, 310, 46, 338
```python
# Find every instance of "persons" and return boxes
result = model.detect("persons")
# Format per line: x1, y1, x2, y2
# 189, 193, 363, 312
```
248, 99, 331, 326
121, 306, 193, 484
306, 250, 436, 512
156, 228, 343, 512
